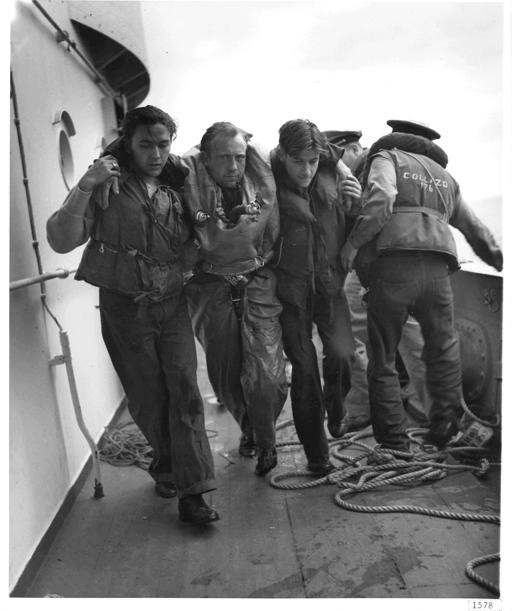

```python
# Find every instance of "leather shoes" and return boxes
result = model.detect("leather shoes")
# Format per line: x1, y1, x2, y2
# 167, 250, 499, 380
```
155, 478, 178, 500
256, 453, 278, 477
327, 411, 351, 439
345, 414, 373, 432
178, 493, 221, 528
238, 433, 258, 459
307, 459, 334, 474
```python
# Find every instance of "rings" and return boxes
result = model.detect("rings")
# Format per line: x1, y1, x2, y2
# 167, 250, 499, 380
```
111, 162, 118, 170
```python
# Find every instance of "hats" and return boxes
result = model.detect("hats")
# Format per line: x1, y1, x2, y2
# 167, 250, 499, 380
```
319, 129, 363, 148
387, 119, 441, 141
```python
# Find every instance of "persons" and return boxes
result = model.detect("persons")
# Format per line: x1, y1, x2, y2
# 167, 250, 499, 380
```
88, 120, 287, 476
340, 118, 503, 466
320, 128, 370, 439
264, 117, 365, 476
43, 103, 222, 526
